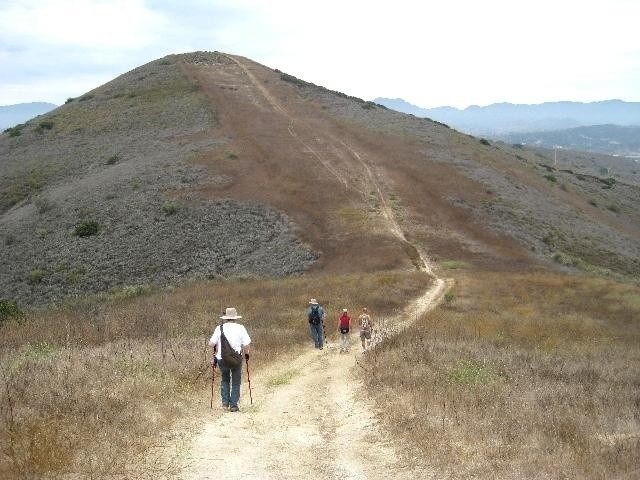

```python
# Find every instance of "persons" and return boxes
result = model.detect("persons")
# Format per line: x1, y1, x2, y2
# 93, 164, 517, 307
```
358, 308, 371, 349
337, 309, 351, 353
308, 298, 326, 350
208, 308, 252, 412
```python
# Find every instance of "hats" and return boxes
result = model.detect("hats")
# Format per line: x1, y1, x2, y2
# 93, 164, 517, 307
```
221, 308, 242, 320
309, 299, 318, 304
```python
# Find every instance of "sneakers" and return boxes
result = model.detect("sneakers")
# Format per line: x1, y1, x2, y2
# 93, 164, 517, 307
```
230, 405, 239, 412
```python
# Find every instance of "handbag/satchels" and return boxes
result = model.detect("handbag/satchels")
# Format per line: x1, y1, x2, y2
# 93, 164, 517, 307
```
340, 328, 349, 334
220, 334, 242, 368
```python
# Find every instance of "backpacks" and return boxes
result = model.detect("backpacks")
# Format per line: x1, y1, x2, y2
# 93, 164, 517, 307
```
309, 306, 320, 325
